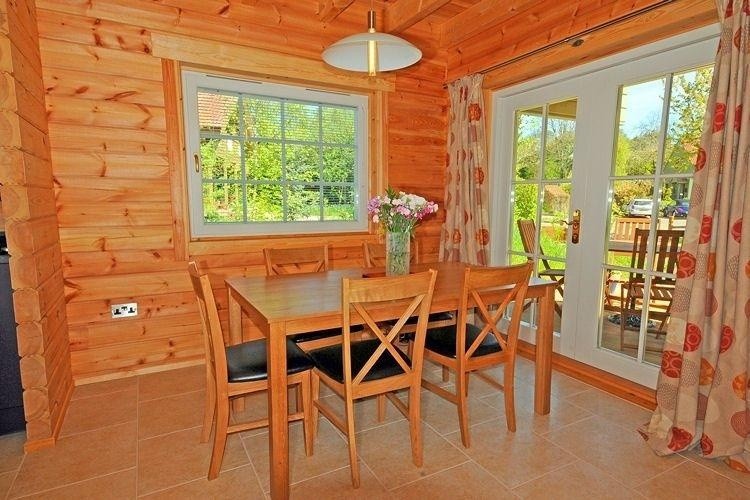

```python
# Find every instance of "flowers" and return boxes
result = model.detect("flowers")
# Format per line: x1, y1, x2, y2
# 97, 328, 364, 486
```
367, 184, 439, 231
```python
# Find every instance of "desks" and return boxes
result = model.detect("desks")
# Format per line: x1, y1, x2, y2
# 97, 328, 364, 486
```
224, 261, 559, 416
604, 241, 682, 328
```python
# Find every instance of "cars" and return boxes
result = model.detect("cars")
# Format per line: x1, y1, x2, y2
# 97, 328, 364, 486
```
626, 199, 654, 216
662, 199, 692, 216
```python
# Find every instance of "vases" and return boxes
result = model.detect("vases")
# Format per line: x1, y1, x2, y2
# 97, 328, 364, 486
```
386, 232, 412, 276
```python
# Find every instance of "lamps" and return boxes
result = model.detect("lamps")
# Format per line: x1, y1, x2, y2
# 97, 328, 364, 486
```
322, 9, 423, 77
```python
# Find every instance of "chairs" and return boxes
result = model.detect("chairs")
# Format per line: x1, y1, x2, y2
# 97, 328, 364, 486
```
408, 261, 534, 449
620, 228, 685, 353
306, 268, 440, 489
187, 261, 315, 481
517, 219, 612, 319
263, 243, 364, 343
363, 238, 458, 381
612, 218, 661, 303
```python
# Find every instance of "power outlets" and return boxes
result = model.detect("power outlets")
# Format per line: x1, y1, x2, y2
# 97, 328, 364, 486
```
111, 303, 138, 318
